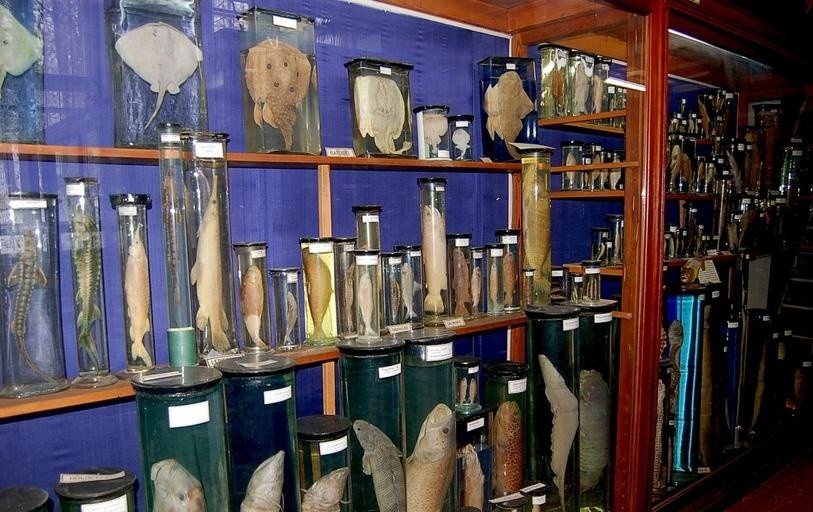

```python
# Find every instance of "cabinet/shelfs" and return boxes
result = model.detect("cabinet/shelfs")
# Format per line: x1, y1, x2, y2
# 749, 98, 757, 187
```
0, 0, 653, 512
517, 0, 813, 512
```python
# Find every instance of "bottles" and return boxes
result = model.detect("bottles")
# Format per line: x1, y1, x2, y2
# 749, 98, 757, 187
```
453, 353, 531, 512
0, 1, 46, 143
103, 0, 213, 149
63, 174, 120, 387
402, 326, 460, 511
132, 351, 300, 511
1, 190, 70, 398
537, 40, 813, 511
1, 486, 52, 509
232, 240, 277, 356
475, 55, 539, 165
51, 464, 139, 512
445, 113, 474, 161
268, 266, 301, 351
348, 54, 416, 162
224, 6, 323, 155
107, 192, 159, 371
300, 176, 536, 347
416, 101, 454, 163
329, 329, 406, 512
291, 414, 354, 510
155, 121, 249, 371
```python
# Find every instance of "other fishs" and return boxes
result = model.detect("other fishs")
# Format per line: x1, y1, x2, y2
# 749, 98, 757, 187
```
408, 44, 813, 512
123, 219, 154, 368
149, 458, 208, 511
240, 263, 268, 350
239, 448, 289, 512
190, 174, 233, 353
72, 207, 107, 375
163, 167, 189, 305
355, 270, 378, 338
489, 263, 504, 315
390, 274, 401, 323
398, 261, 422, 320
284, 291, 299, 345
302, 245, 333, 342
300, 467, 350, 512
4, 224, 59, 389
352, 416, 408, 512
